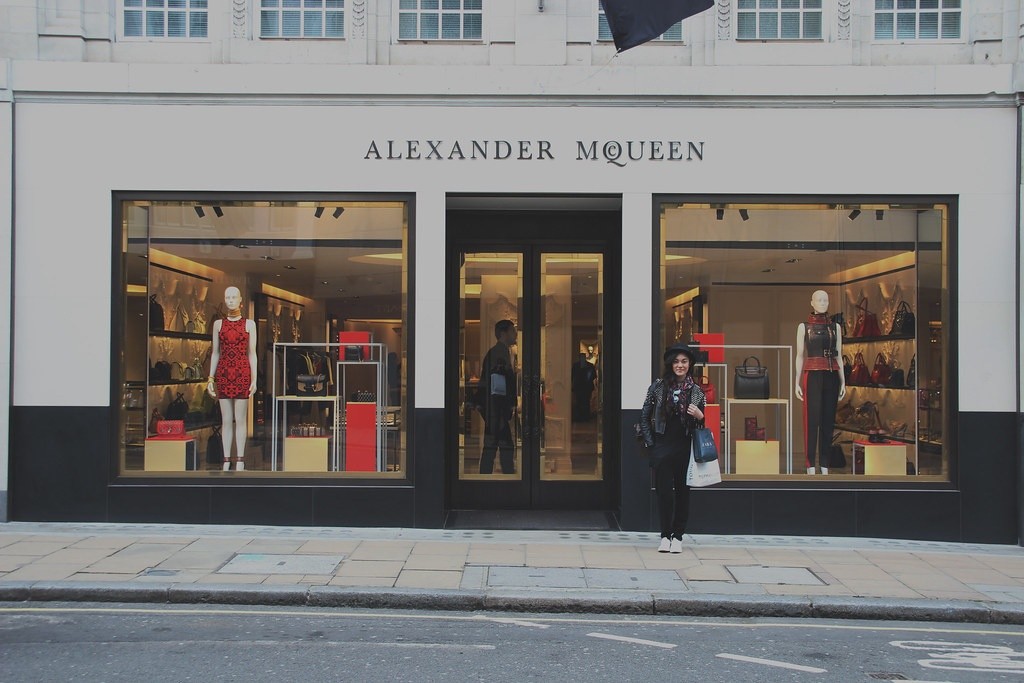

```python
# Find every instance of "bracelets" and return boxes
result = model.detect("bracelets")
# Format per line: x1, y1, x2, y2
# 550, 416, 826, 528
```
206, 375, 215, 384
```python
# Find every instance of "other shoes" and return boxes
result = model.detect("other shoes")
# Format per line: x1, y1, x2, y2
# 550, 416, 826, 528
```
657, 537, 683, 553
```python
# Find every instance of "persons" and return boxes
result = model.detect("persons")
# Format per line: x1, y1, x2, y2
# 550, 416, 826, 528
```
585, 346, 600, 410
637, 342, 707, 553
568, 352, 597, 421
205, 287, 258, 470
474, 318, 520, 474
794, 289, 848, 475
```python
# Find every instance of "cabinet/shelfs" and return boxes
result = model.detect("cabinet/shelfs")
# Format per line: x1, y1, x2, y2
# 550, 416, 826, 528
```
678, 248, 919, 476
147, 242, 405, 472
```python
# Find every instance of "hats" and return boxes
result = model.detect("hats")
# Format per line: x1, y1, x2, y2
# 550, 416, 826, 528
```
664, 343, 696, 367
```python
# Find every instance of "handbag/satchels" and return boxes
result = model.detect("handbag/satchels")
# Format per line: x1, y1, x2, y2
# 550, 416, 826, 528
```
351, 391, 376, 402
698, 376, 715, 404
166, 389, 222, 428
157, 420, 186, 439
733, 356, 769, 399
685, 418, 723, 488
296, 373, 327, 397
692, 418, 718, 462
744, 416, 766, 441
148, 408, 166, 433
290, 422, 326, 436
688, 340, 709, 365
829, 294, 915, 475
125, 388, 151, 411
344, 345, 365, 362
150, 293, 227, 382
205, 424, 225, 464
